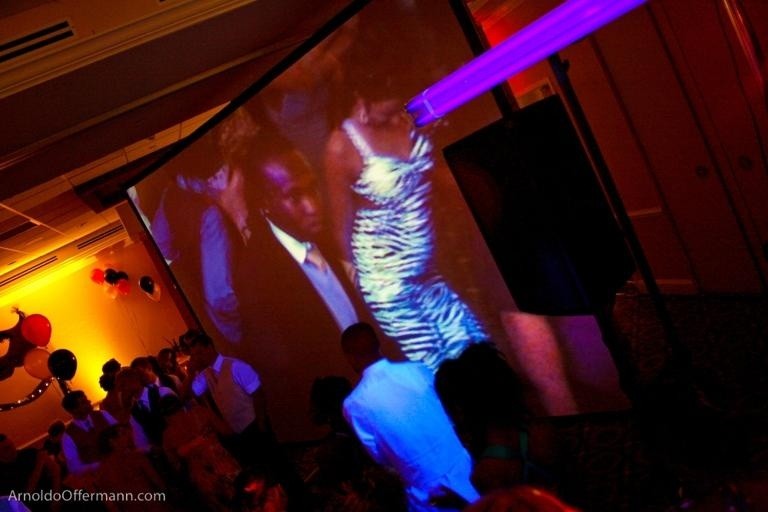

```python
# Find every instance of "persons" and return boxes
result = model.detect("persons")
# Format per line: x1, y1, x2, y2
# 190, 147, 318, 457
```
128, 36, 495, 376
1, 321, 575, 511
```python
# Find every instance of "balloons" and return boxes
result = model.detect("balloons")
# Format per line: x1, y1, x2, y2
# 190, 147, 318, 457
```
20, 312, 53, 350
88, 266, 164, 303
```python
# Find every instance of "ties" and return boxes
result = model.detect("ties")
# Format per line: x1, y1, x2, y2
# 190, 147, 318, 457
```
140, 400, 149, 412
306, 243, 328, 274
209, 368, 218, 384
84, 421, 92, 430
153, 384, 158, 391
